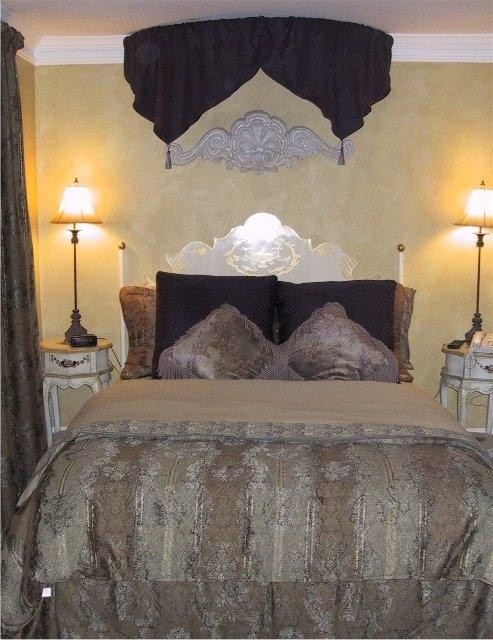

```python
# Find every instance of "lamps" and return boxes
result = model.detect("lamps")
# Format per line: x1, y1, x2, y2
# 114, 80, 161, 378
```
454, 181, 493, 345
51, 177, 102, 347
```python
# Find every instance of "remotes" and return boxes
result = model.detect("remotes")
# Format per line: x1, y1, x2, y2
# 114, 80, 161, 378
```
447, 340, 463, 349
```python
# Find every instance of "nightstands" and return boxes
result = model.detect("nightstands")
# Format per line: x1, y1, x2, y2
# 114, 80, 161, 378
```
439, 343, 493, 438
37, 337, 114, 450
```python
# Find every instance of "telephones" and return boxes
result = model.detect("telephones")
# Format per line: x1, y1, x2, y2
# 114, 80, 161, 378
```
471, 331, 493, 354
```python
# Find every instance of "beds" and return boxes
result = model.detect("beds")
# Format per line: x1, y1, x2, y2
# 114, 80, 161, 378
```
1, 212, 493, 638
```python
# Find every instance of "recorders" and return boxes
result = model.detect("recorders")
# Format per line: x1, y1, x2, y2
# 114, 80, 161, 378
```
70, 334, 97, 347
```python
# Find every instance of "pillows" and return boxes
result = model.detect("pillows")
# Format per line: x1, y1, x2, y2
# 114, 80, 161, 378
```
152, 272, 277, 380
274, 279, 396, 353
157, 304, 292, 380
119, 286, 156, 379
395, 283, 417, 382
280, 302, 399, 383
